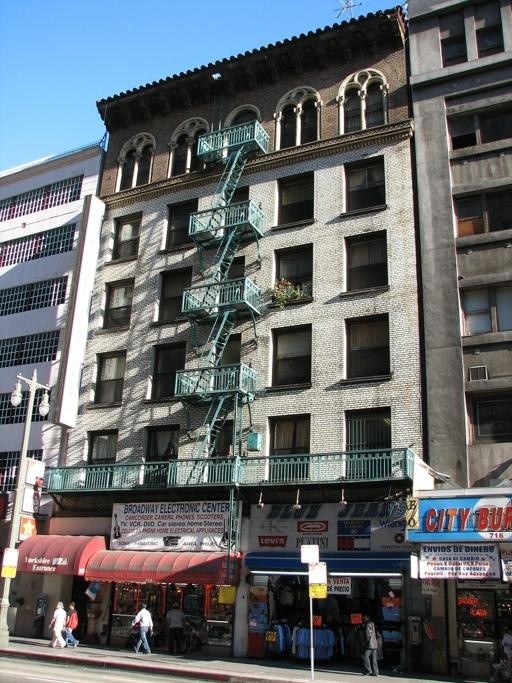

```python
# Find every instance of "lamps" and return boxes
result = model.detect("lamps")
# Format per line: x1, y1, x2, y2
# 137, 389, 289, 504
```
257, 492, 264, 509
293, 489, 301, 510
339, 489, 347, 506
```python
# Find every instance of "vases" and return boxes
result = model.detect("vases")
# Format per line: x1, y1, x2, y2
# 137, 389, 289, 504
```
271, 296, 277, 302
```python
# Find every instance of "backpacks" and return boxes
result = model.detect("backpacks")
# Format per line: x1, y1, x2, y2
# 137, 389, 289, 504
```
68, 610, 79, 629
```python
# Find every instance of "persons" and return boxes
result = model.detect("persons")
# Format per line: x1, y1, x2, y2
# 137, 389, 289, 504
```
132, 603, 155, 655
166, 603, 186, 654
49, 600, 68, 648
65, 601, 80, 647
360, 613, 379, 677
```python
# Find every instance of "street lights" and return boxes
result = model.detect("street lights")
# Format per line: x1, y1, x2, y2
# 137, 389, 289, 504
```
0, 369, 52, 647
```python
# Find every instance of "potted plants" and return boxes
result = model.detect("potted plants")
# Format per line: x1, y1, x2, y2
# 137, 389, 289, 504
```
290, 290, 304, 299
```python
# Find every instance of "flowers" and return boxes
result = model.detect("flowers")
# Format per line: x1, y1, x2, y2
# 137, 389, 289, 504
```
269, 278, 291, 311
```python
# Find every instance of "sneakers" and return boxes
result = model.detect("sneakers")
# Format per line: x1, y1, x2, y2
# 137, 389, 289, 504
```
49, 639, 80, 648
134, 647, 150, 654
363, 669, 378, 676
